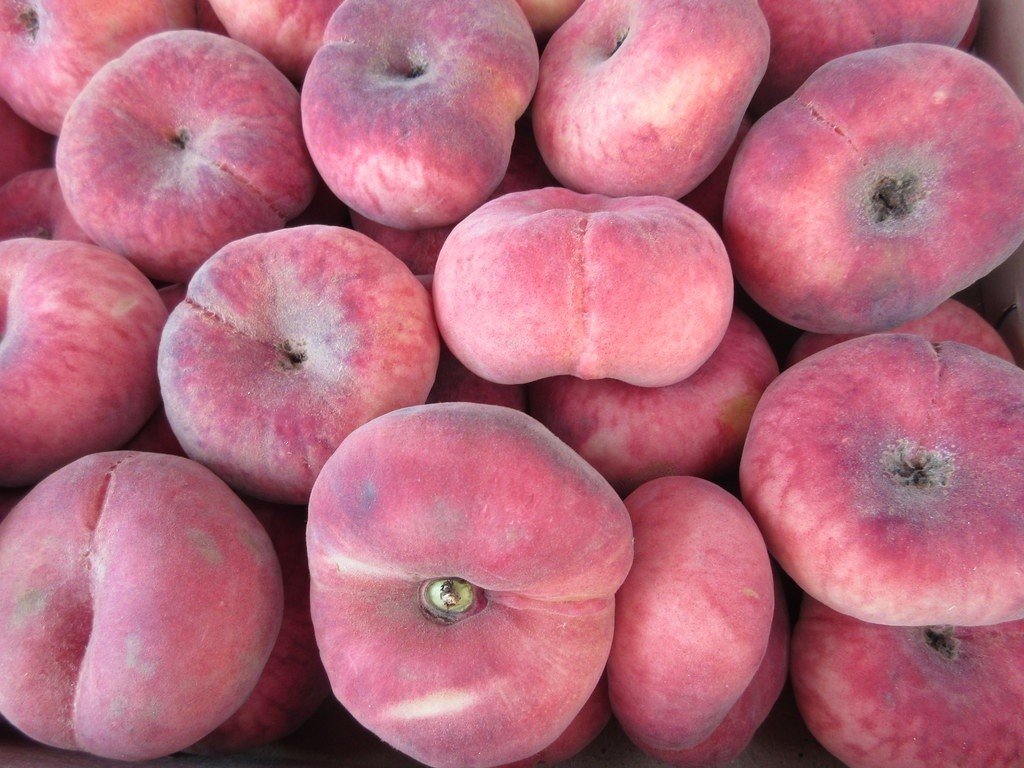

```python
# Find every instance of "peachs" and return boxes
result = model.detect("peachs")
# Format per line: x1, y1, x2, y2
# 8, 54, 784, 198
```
0, 0, 1024, 768
